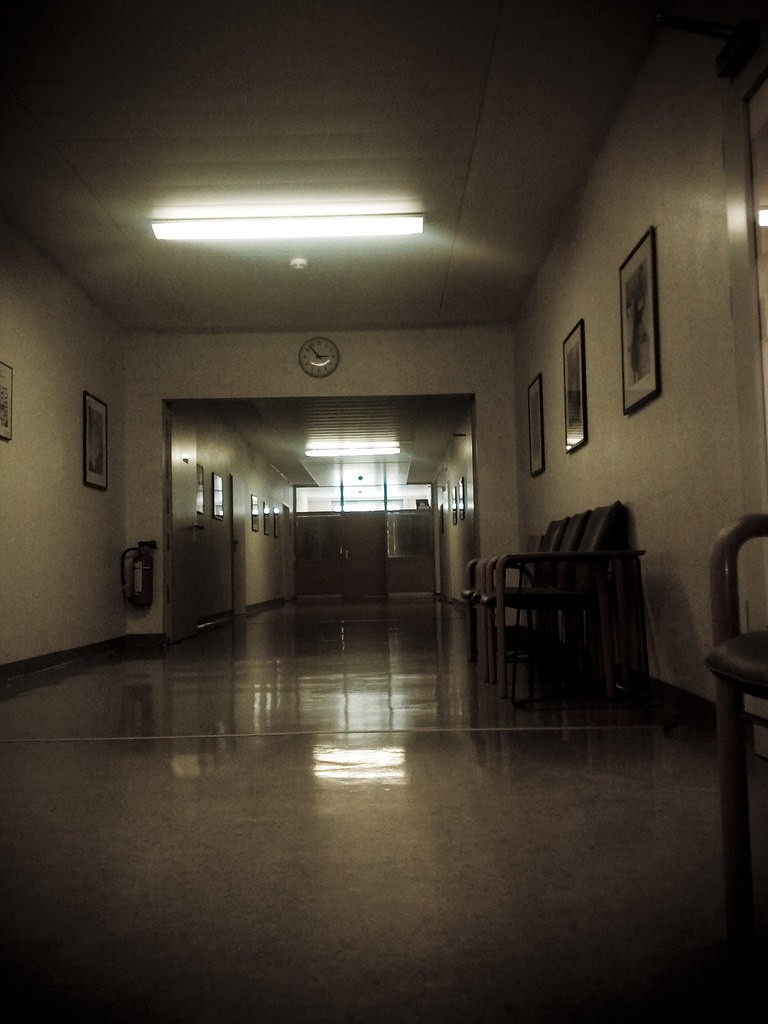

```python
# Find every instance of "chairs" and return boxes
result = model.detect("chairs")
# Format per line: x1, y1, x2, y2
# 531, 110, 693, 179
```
702, 516, 768, 946
461, 500, 646, 700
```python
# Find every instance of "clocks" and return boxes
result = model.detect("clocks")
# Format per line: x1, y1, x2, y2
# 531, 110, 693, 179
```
298, 337, 340, 378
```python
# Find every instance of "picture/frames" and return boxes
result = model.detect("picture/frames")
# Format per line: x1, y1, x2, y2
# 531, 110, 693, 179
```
82, 390, 108, 491
212, 472, 224, 521
0, 361, 13, 441
527, 372, 546, 478
619, 225, 661, 415
562, 319, 588, 455
452, 478, 465, 525
196, 463, 204, 515
263, 501, 279, 538
251, 494, 259, 531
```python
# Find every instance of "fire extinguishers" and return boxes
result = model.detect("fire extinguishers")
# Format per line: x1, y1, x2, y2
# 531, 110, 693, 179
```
121, 541, 158, 607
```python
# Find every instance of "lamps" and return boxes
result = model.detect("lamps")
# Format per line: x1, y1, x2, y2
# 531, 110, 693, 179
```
152, 213, 425, 240
305, 448, 400, 457
